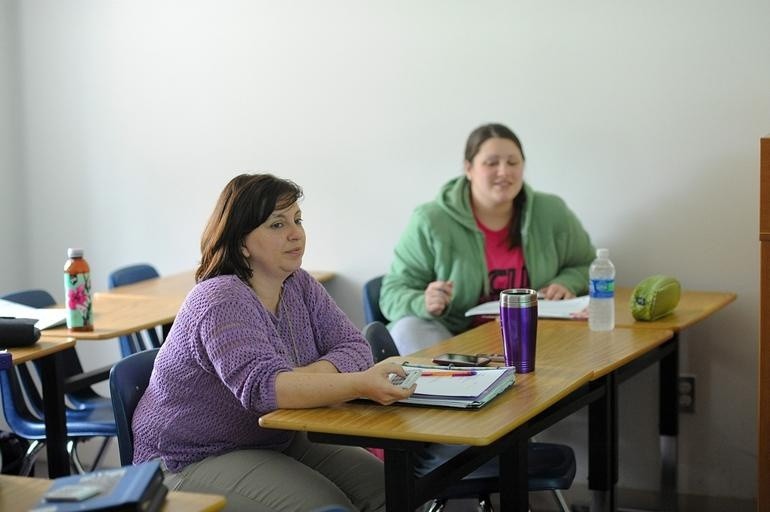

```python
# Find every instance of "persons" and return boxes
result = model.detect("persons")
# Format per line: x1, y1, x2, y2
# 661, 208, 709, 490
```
378, 125, 596, 357
131, 174, 417, 512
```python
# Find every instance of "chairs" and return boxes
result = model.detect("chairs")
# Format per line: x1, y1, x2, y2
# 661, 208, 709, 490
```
362, 321, 576, 510
2, 266, 388, 478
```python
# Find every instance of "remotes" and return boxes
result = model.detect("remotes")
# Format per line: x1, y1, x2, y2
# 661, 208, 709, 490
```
387, 367, 422, 390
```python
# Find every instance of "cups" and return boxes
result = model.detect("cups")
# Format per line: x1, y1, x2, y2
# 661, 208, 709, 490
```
499, 287, 539, 374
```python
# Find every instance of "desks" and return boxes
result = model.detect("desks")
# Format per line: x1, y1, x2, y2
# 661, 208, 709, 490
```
1, 475, 227, 511
260, 287, 737, 508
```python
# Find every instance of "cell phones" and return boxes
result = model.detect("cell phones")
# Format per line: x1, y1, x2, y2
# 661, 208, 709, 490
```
432, 352, 491, 366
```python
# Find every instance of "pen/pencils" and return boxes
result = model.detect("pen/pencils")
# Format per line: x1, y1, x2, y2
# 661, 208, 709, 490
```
421, 371, 476, 377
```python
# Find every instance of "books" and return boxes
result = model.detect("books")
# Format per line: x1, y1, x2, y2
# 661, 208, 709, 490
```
29, 459, 168, 512
349, 361, 516, 410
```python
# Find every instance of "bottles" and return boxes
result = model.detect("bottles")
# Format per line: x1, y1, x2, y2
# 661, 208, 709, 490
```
589, 248, 616, 333
63, 248, 94, 333
